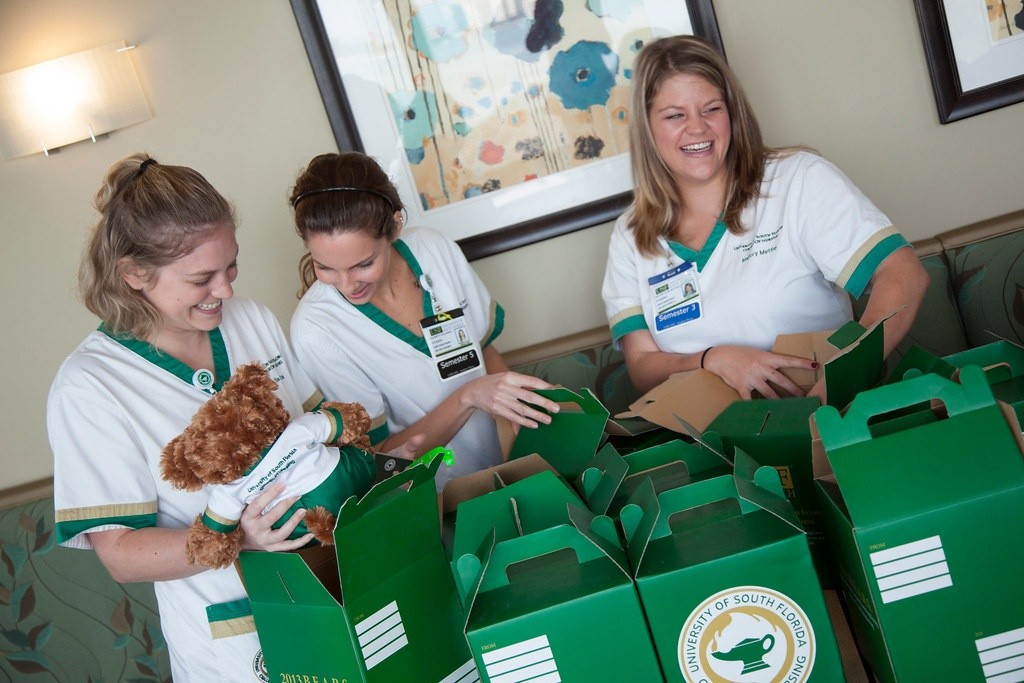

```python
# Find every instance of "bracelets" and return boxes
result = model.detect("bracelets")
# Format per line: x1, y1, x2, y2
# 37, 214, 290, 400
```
701, 347, 714, 369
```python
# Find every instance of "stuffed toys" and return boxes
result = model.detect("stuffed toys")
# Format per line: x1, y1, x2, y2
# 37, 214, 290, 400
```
160, 364, 378, 568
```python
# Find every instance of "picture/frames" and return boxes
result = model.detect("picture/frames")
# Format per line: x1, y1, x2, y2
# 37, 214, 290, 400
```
288, 1, 727, 263
912, 0, 1024, 125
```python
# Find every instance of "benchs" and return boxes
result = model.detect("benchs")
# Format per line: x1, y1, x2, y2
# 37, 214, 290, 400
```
0, 208, 1024, 683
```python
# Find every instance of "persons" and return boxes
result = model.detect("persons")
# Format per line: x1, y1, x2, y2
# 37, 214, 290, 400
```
290, 153, 560, 493
47, 154, 428, 682
684, 283, 696, 297
601, 35, 932, 407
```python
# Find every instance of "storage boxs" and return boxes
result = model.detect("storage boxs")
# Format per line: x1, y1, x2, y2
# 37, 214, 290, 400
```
241, 313, 1024, 683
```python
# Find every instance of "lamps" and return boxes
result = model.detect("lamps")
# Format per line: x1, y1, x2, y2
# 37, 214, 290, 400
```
0, 42, 154, 164
